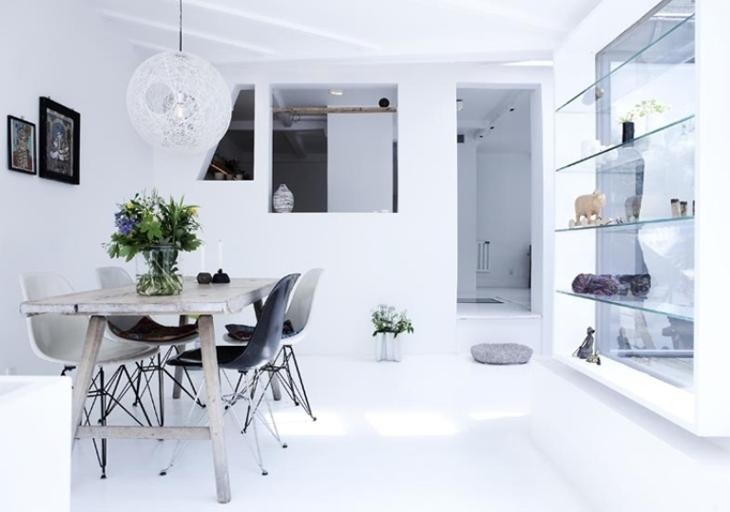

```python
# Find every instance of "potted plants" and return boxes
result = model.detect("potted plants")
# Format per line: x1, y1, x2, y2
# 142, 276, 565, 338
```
619, 98, 671, 143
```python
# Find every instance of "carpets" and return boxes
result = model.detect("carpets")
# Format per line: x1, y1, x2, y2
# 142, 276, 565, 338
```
457, 298, 503, 303
473, 344, 531, 365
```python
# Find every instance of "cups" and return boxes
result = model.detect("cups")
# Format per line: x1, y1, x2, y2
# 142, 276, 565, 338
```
226, 174, 233, 180
214, 172, 224, 180
236, 173, 243, 180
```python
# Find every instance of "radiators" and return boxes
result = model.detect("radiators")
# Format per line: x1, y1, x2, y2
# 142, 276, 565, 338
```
477, 240, 491, 272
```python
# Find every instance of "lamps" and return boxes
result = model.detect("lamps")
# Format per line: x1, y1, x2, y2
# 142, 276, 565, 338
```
125, 0, 232, 154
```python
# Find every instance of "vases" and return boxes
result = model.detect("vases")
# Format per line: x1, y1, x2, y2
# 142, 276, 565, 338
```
136, 244, 183, 295
273, 184, 294, 212
376, 333, 402, 362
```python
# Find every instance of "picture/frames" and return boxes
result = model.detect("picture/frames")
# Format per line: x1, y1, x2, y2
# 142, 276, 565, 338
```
6, 114, 38, 174
38, 95, 80, 185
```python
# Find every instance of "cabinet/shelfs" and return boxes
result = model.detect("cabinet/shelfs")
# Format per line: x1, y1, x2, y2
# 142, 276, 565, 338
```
554, 3, 728, 439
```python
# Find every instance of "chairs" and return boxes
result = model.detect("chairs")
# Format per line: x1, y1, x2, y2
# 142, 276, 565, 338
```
160, 272, 301, 477
95, 267, 206, 409
225, 267, 323, 421
19, 273, 164, 479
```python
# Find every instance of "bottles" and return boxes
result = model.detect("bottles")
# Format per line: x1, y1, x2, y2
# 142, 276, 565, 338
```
680, 201, 688, 217
670, 198, 681, 217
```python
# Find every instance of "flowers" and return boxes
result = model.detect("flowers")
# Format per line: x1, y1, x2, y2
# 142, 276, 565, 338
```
370, 304, 414, 337
102, 189, 206, 292
